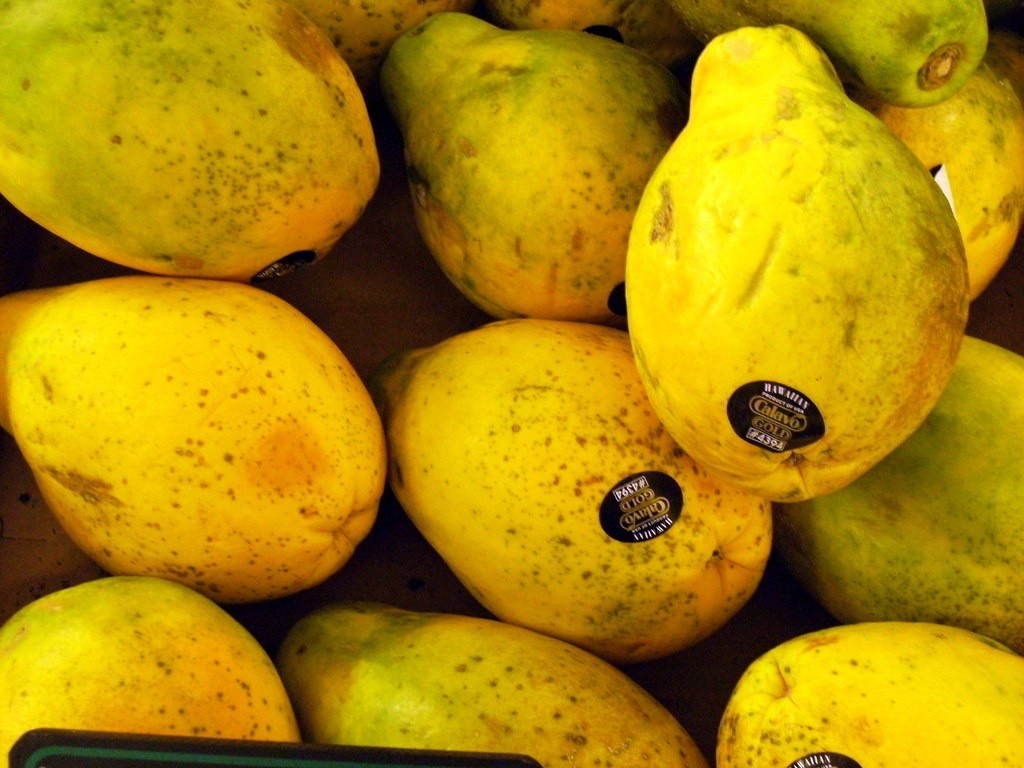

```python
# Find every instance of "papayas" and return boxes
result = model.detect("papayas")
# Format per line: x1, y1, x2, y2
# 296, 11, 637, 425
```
0, 0, 1024, 768
